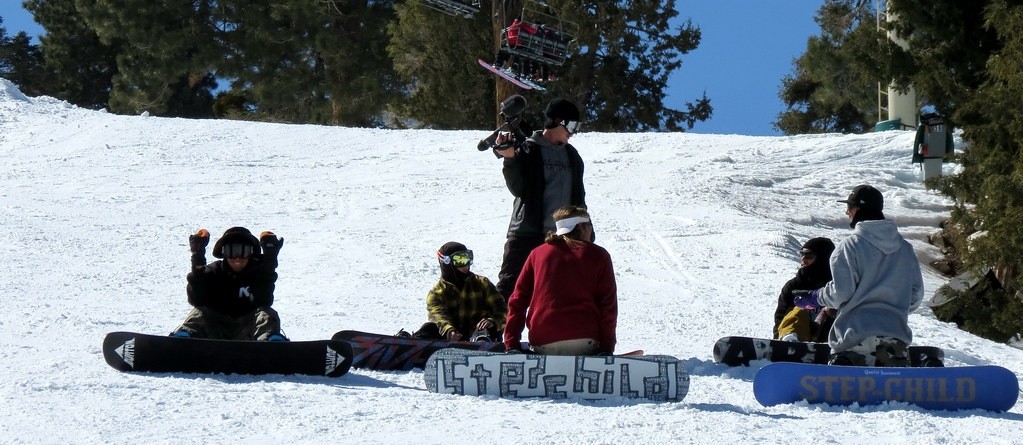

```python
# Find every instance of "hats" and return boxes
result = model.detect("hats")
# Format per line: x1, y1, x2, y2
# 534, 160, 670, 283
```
213, 227, 262, 258
800, 237, 836, 259
545, 98, 583, 125
555, 216, 590, 236
836, 184, 883, 211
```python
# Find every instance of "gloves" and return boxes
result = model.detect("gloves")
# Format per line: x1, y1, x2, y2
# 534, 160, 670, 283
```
260, 230, 284, 266
189, 229, 210, 266
791, 288, 822, 310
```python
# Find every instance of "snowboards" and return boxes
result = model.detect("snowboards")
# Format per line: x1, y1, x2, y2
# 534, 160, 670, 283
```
101, 330, 354, 378
331, 329, 530, 371
424, 347, 691, 404
753, 362, 1020, 411
477, 58, 547, 93
713, 336, 944, 368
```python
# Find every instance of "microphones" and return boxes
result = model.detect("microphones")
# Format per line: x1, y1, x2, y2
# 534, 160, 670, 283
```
499, 94, 527, 118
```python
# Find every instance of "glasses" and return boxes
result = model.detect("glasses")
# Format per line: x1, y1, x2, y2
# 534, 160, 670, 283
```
450, 250, 474, 268
800, 250, 815, 259
222, 243, 253, 258
847, 203, 858, 211
565, 120, 582, 134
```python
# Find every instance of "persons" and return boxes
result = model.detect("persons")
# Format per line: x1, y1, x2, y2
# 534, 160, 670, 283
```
494, 97, 596, 304
792, 184, 924, 367
504, 206, 618, 355
168, 226, 290, 342
492, 19, 567, 85
774, 237, 838, 343
395, 241, 508, 343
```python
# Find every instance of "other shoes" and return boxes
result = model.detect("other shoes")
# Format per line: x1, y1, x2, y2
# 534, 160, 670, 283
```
495, 64, 500, 70
